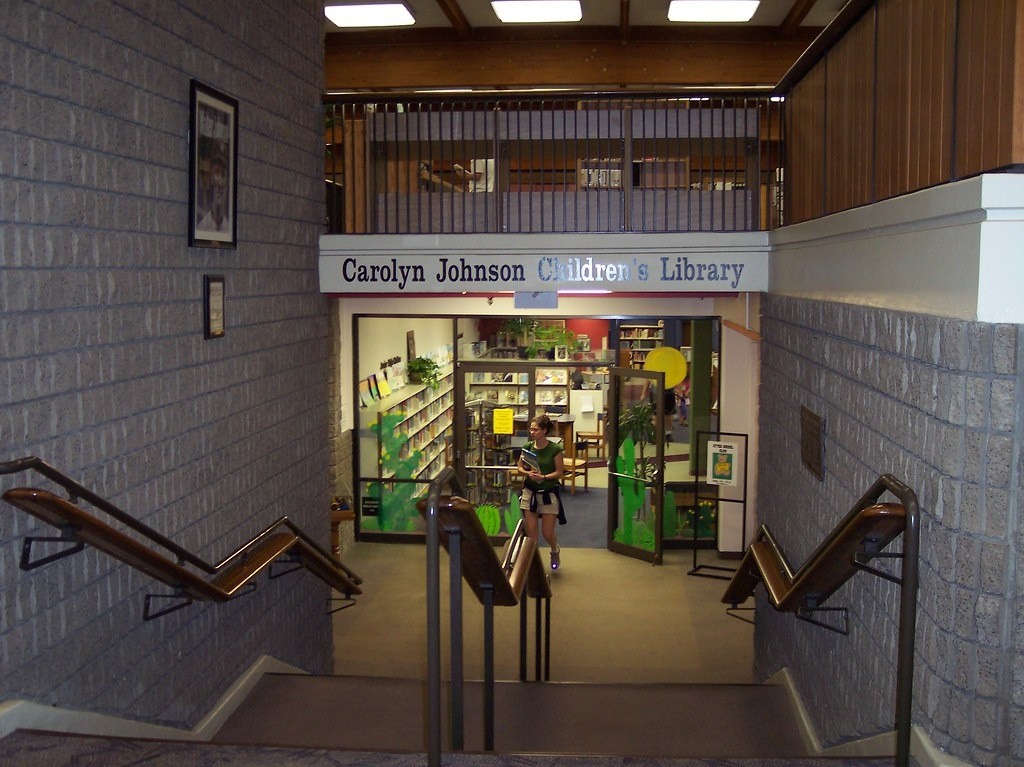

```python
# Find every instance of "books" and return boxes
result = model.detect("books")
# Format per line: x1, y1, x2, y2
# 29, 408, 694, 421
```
630, 362, 644, 370
629, 352, 646, 360
521, 448, 543, 476
381, 388, 453, 453
411, 447, 453, 497
417, 343, 454, 370
655, 341, 661, 348
513, 407, 563, 419
518, 371, 564, 385
465, 407, 529, 505
520, 389, 566, 404
381, 406, 453, 478
471, 388, 517, 404
619, 340, 638, 348
380, 372, 453, 428
473, 371, 513, 384
619, 328, 663, 339
462, 330, 595, 361
383, 425, 454, 491
358, 361, 408, 409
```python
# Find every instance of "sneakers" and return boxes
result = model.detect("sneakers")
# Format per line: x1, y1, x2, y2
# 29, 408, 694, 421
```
550, 546, 560, 569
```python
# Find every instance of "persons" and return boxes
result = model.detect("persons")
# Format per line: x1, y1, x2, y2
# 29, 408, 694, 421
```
571, 357, 602, 390
641, 372, 689, 442
518, 415, 563, 569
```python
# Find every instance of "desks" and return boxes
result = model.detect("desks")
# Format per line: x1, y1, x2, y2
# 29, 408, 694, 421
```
511, 436, 562, 450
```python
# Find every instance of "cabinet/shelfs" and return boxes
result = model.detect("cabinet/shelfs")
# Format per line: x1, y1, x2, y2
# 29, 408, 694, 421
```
360, 348, 570, 531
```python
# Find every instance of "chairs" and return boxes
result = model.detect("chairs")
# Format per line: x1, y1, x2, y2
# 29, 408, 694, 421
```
562, 438, 588, 495
577, 413, 605, 464
508, 451, 525, 485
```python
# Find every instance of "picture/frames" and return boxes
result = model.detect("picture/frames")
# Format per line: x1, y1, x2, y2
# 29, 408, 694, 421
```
204, 274, 226, 339
186, 79, 239, 251
534, 320, 565, 343
